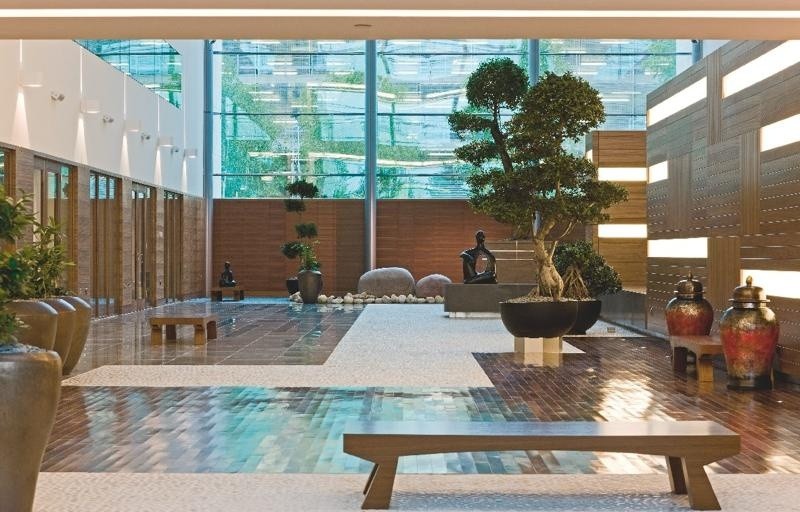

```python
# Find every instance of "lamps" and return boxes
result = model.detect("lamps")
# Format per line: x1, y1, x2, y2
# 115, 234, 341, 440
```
123, 118, 139, 132
158, 135, 176, 146
184, 148, 198, 158
80, 98, 101, 112
16, 66, 44, 87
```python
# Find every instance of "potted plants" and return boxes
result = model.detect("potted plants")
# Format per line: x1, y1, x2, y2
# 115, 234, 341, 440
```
447, 57, 629, 337
546, 238, 626, 334
278, 179, 324, 304
0, 182, 93, 512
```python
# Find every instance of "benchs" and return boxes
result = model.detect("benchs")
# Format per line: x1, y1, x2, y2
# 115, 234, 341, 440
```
670, 334, 775, 383
337, 419, 741, 510
149, 314, 218, 346
208, 287, 245, 302
